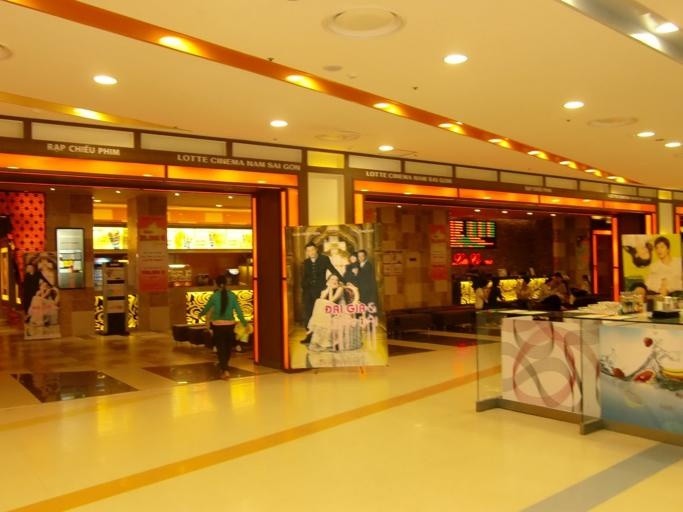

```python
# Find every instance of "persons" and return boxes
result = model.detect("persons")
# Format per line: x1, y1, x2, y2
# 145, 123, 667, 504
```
20, 262, 54, 323
24, 280, 58, 329
623, 235, 655, 268
196, 273, 249, 381
342, 251, 361, 287
351, 248, 376, 332
306, 273, 354, 354
644, 236, 683, 294
299, 241, 352, 345
630, 282, 649, 300
453, 264, 594, 311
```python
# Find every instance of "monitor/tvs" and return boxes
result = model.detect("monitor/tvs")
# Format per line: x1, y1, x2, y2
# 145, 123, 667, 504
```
225, 269, 240, 285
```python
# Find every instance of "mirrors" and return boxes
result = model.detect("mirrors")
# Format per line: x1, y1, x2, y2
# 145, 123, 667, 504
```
55, 227, 86, 289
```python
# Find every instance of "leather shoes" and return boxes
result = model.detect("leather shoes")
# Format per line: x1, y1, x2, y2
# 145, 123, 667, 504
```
301, 332, 313, 343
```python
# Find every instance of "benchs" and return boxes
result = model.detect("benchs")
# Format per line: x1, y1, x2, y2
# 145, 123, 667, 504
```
385, 287, 599, 339
172, 319, 255, 353
